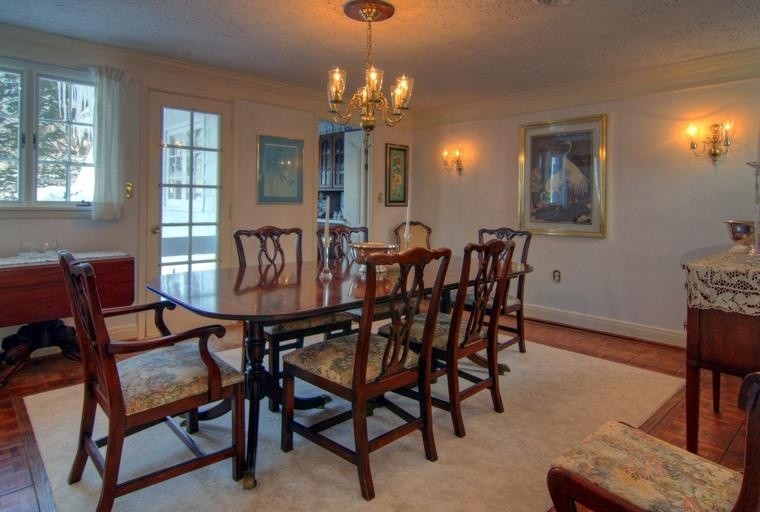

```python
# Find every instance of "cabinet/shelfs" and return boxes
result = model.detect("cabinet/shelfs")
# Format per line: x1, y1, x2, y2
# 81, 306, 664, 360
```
319, 132, 344, 189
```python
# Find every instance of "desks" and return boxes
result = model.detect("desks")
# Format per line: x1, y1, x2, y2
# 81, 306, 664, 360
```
680, 240, 760, 454
0, 251, 135, 382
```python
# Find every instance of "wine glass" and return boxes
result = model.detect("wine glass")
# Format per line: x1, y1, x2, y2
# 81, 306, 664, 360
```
347, 270, 396, 300
348, 241, 399, 273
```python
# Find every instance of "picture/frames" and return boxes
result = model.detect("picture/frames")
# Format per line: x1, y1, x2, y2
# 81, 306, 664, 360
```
519, 113, 608, 238
385, 143, 409, 206
257, 134, 304, 204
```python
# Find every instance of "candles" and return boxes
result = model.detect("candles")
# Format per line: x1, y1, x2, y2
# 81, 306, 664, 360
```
325, 196, 330, 237
404, 200, 411, 240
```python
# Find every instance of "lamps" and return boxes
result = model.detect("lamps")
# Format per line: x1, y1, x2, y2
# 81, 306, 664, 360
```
441, 149, 462, 176
685, 119, 737, 165
327, 0, 414, 131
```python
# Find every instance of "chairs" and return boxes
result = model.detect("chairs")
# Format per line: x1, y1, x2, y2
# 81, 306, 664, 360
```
547, 373, 760, 512
281, 248, 451, 500
315, 225, 418, 340
377, 240, 514, 439
233, 225, 352, 411
58, 252, 244, 512
396, 221, 432, 252
441, 227, 532, 352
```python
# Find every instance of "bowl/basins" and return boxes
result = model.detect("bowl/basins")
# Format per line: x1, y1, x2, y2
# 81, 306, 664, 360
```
722, 219, 756, 244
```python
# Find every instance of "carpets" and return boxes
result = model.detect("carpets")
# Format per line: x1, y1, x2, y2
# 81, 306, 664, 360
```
11, 317, 686, 512
